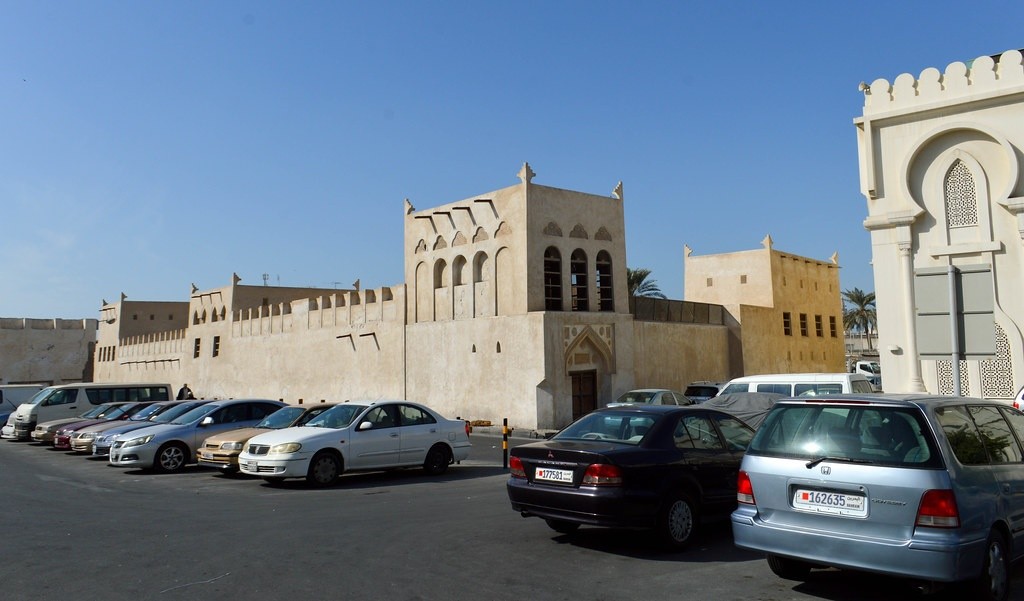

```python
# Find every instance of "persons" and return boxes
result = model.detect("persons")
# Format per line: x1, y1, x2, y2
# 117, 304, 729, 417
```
175, 383, 194, 400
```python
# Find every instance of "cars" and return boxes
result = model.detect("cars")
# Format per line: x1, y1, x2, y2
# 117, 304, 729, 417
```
29, 409, 100, 444
53, 402, 130, 448
236, 399, 472, 487
0, 411, 23, 440
68, 401, 163, 453
196, 402, 382, 476
676, 392, 851, 449
107, 398, 291, 472
603, 388, 702, 437
504, 404, 778, 546
92, 399, 200, 457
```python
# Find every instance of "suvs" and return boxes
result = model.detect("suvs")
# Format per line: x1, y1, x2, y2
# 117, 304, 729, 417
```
729, 394, 1024, 601
682, 380, 731, 405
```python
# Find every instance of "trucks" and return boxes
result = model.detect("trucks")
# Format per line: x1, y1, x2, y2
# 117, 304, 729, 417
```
847, 360, 882, 390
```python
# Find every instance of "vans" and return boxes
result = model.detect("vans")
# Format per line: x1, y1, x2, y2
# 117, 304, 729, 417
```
715, 372, 884, 430
0, 384, 45, 431
12, 383, 174, 437
